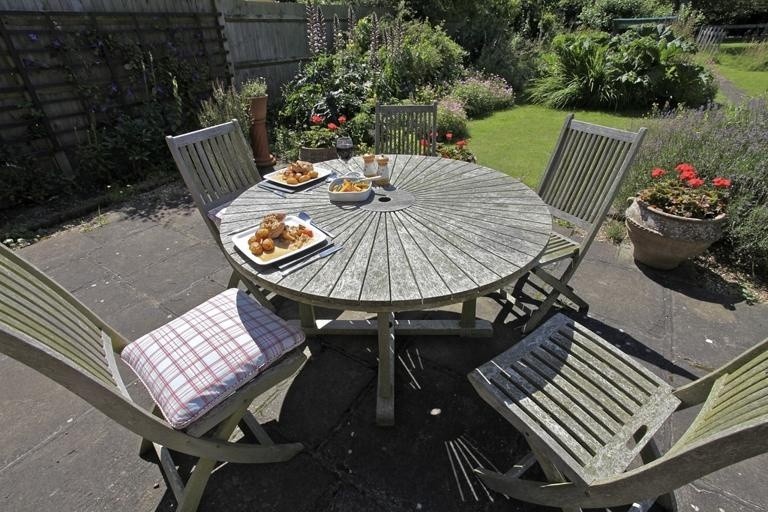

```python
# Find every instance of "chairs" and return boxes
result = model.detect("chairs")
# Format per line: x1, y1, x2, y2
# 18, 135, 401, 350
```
1, 241, 310, 510
491, 113, 648, 338
468, 312, 763, 510
165, 121, 304, 312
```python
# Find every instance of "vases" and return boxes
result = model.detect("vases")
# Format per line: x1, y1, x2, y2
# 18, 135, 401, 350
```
297, 136, 340, 161
618, 190, 728, 269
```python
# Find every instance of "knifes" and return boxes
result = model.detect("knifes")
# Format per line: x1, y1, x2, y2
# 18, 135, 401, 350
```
281, 244, 343, 277
258, 184, 287, 199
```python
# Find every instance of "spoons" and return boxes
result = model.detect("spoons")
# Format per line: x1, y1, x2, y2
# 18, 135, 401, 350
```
299, 211, 335, 239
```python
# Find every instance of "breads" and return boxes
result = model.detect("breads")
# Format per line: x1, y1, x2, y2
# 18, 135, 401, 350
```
290, 160, 313, 173
259, 214, 285, 239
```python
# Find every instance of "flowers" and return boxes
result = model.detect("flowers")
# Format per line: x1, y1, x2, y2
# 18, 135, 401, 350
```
305, 110, 347, 132
642, 158, 733, 196
462, 71, 513, 119
420, 128, 476, 161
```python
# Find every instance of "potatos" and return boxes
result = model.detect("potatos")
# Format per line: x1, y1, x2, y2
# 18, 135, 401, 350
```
287, 171, 318, 184
249, 228, 273, 255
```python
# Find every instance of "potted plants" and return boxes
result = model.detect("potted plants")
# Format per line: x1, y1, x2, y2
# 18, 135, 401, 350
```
243, 75, 275, 165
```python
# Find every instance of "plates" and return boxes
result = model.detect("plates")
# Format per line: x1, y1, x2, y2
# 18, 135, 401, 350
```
328, 177, 372, 201
262, 165, 331, 189
233, 216, 325, 264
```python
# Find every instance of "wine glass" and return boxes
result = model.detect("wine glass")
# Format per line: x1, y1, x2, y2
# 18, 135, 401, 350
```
337, 138, 353, 174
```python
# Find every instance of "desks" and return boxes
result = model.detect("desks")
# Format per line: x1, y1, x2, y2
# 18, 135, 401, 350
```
216, 152, 556, 430
